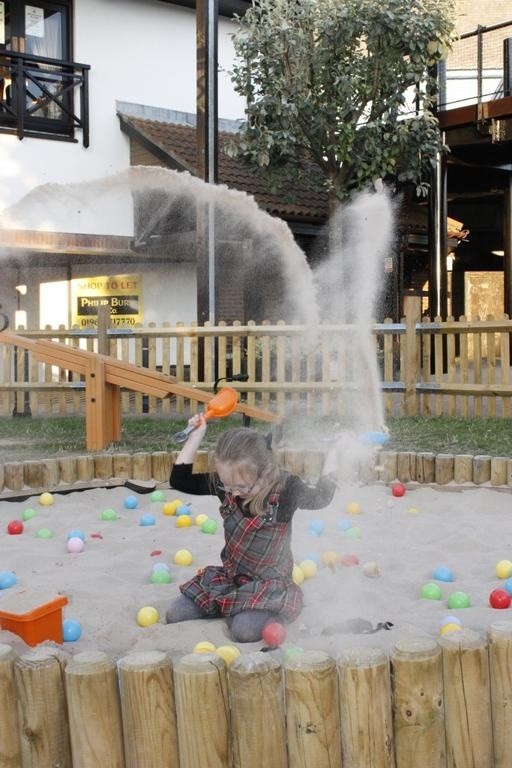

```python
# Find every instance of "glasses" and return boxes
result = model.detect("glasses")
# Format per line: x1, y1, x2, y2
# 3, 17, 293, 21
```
218, 474, 259, 493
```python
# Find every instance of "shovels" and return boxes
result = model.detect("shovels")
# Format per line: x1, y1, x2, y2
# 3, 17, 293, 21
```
172, 387, 239, 444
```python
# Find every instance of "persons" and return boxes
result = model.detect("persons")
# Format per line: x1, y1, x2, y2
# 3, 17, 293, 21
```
165, 412, 348, 643
